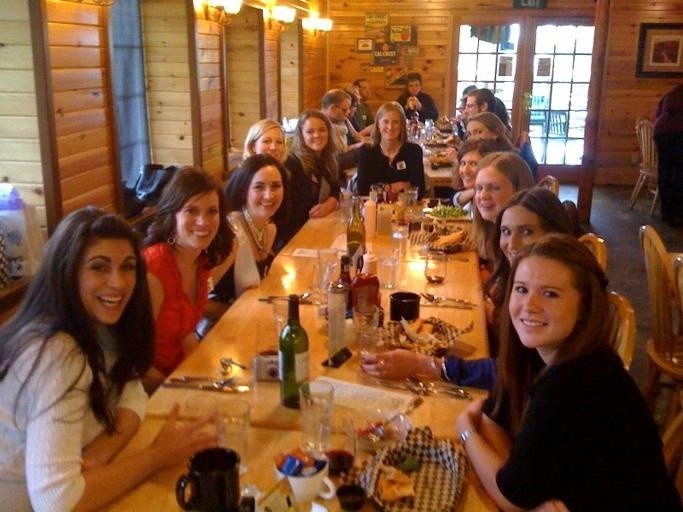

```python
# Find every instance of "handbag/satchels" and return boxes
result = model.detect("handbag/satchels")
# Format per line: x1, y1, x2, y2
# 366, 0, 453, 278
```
134, 162, 177, 206
122, 181, 143, 218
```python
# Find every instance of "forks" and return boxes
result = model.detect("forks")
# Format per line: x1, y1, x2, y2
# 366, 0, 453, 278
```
419, 292, 477, 309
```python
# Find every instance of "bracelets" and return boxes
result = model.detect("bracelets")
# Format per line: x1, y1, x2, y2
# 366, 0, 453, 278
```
430, 357, 449, 381
459, 427, 476, 441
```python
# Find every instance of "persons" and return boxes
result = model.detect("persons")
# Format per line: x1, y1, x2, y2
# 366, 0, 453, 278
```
396, 72, 439, 125
340, 99, 426, 200
455, 233, 683, 511
209, 77, 375, 302
435, 86, 540, 335
652, 84, 683, 232
358, 186, 592, 392
378, 42, 393, 62
0, 203, 223, 511
139, 164, 236, 388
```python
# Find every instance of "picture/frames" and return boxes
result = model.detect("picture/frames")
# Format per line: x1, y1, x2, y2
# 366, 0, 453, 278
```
633, 21, 682, 78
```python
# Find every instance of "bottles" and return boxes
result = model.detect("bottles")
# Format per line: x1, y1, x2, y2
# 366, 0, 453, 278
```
279, 295, 310, 408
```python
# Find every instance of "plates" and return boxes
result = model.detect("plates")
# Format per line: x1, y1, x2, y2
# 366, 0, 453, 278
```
423, 207, 472, 221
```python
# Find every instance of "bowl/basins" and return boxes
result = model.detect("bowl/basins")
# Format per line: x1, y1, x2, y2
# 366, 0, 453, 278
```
337, 484, 368, 509
342, 407, 411, 453
327, 449, 354, 476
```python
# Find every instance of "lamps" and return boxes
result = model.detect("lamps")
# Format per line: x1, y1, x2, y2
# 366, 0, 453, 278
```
204, 1, 242, 26
302, 14, 335, 39
273, 5, 294, 35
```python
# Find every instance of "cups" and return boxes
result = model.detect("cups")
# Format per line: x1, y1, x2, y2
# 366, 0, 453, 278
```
424, 251, 445, 283
272, 297, 291, 343
406, 119, 435, 160
310, 295, 390, 384
339, 187, 418, 238
275, 453, 336, 502
183, 394, 250, 475
317, 247, 400, 290
298, 379, 337, 451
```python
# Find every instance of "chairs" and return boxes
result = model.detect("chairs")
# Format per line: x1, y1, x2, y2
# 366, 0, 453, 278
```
629, 114, 648, 206
600, 286, 637, 370
673, 257, 682, 303
536, 173, 565, 200
660, 408, 683, 511
629, 119, 656, 216
578, 232, 611, 271
640, 224, 678, 431
